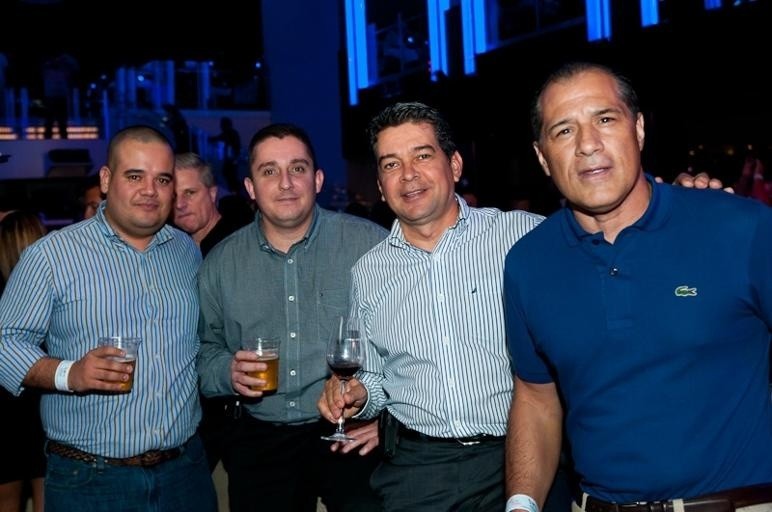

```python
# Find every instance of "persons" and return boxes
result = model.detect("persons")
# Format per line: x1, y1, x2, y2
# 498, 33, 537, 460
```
501, 55, 771, 512
169, 151, 241, 511
1, 210, 47, 511
317, 98, 739, 512
0, 123, 220, 510
195, 121, 392, 512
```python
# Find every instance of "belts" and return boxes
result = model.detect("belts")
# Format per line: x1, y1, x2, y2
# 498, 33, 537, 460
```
398, 422, 507, 448
46, 436, 190, 468
573, 486, 771, 511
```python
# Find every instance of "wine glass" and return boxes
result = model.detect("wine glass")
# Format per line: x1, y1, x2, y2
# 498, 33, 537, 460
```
322, 318, 367, 442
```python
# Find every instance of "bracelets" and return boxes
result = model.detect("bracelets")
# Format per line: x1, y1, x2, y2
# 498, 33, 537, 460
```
504, 493, 539, 512
54, 358, 75, 395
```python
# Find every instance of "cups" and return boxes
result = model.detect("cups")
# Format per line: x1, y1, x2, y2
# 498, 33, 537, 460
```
91, 337, 140, 393
244, 336, 279, 392
